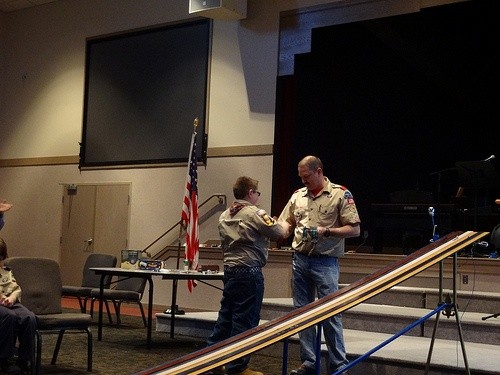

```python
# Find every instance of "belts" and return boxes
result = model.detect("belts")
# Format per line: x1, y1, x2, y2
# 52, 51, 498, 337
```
223, 266, 262, 273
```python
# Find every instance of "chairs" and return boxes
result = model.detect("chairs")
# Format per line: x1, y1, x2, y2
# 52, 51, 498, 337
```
0, 257, 92, 375
62, 254, 117, 323
88, 257, 150, 328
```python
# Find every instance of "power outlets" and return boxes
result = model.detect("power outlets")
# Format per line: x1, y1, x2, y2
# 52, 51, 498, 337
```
463, 274, 468, 284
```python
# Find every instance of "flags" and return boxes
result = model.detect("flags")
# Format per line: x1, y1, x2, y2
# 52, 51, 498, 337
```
180, 131, 200, 293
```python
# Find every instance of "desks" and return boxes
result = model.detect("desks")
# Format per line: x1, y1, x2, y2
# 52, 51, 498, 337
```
89, 267, 224, 351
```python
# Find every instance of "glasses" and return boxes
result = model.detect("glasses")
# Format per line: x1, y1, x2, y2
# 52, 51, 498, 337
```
253, 191, 261, 196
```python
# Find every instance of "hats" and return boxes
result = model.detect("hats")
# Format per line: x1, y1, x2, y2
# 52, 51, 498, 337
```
0, 202, 12, 212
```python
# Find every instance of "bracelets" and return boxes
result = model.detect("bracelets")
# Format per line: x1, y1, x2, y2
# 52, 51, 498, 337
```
324, 227, 331, 238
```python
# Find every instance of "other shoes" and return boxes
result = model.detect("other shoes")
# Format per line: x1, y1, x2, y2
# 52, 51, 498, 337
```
0, 365, 21, 375
236, 368, 263, 375
292, 365, 314, 375
15, 357, 31, 375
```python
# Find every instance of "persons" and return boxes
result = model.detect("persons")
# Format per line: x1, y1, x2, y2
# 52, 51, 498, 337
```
277, 156, 361, 375
0, 238, 37, 375
0, 200, 13, 231
206, 176, 289, 375
